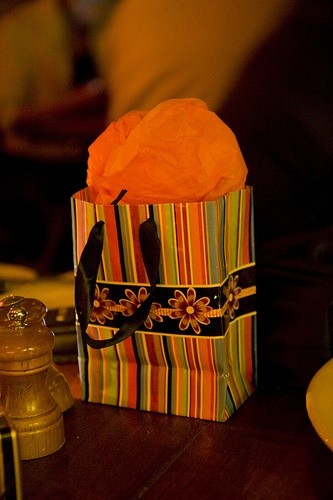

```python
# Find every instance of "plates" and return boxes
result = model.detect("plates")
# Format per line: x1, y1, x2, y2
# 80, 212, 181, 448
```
306, 357, 333, 453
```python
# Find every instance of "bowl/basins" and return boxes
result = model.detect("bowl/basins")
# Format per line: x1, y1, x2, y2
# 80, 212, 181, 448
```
43, 304, 78, 362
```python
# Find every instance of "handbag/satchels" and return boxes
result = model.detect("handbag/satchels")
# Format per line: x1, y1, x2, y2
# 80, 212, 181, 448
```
71, 184, 259, 422
